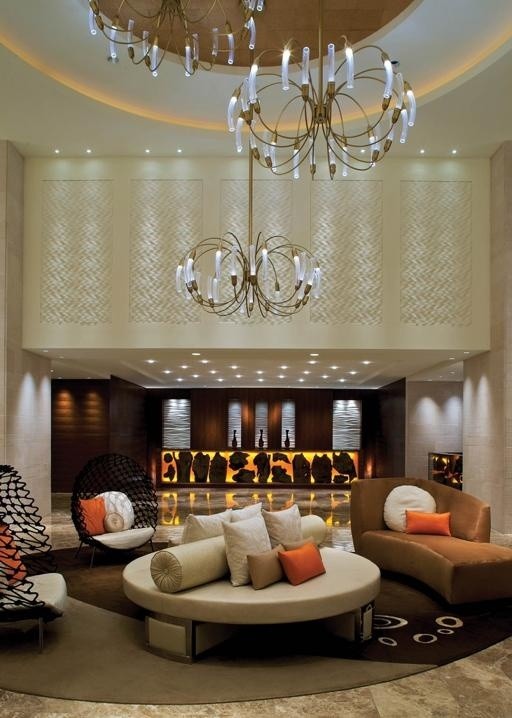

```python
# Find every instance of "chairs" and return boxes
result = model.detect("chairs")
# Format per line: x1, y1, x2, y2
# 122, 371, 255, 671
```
0, 465, 67, 653
70, 452, 159, 568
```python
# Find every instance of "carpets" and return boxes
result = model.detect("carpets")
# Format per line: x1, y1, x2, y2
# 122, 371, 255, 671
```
0, 542, 512, 704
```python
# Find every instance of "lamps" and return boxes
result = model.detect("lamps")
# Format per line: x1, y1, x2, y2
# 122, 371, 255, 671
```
174, 49, 321, 317
227, 1, 418, 180
87, 0, 263, 78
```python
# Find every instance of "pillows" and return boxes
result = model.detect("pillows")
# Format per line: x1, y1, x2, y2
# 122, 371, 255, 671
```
180, 501, 326, 590
0, 525, 27, 591
383, 485, 451, 536
78, 491, 135, 536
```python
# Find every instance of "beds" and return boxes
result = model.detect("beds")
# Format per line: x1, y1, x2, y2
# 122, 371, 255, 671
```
351, 477, 512, 616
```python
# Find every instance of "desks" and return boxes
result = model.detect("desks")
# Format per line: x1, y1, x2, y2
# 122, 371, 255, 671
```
123, 546, 382, 663
